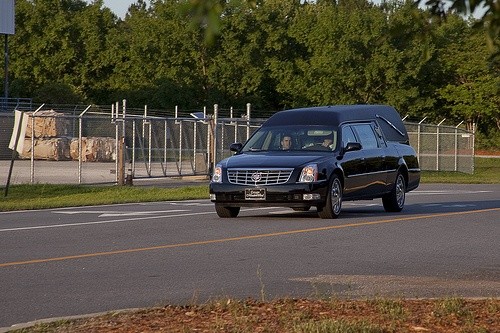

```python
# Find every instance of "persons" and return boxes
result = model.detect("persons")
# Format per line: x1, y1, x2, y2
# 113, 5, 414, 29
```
280, 135, 292, 151
322, 134, 334, 151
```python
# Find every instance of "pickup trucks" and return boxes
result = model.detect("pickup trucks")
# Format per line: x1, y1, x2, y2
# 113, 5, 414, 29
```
209, 105, 422, 219
